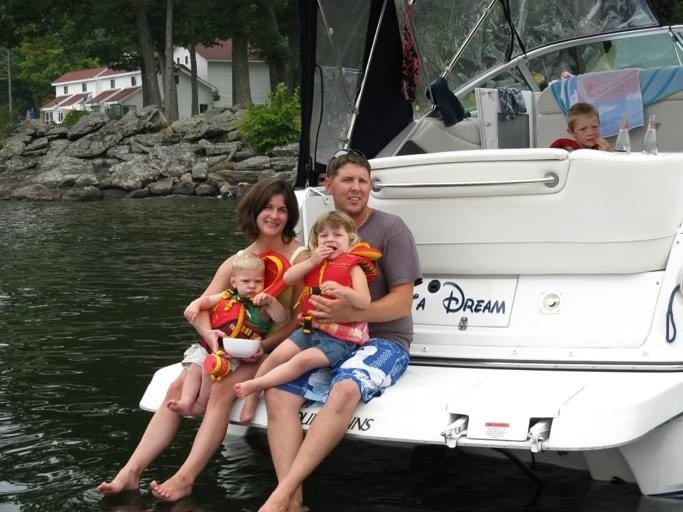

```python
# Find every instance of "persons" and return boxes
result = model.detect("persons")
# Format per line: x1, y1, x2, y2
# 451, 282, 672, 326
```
96, 177, 311, 501
255, 145, 423, 510
549, 101, 616, 153
165, 254, 287, 416
232, 210, 371, 425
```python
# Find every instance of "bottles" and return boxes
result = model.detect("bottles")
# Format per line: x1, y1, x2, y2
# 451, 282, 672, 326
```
615, 111, 631, 152
641, 114, 658, 153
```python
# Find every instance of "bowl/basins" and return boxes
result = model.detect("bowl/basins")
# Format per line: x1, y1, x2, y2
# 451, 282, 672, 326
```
224, 337, 261, 359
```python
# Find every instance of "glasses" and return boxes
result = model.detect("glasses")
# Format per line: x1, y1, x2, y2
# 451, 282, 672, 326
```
327, 149, 369, 176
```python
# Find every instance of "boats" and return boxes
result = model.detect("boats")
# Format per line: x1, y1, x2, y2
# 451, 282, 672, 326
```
139, 0, 682, 496
